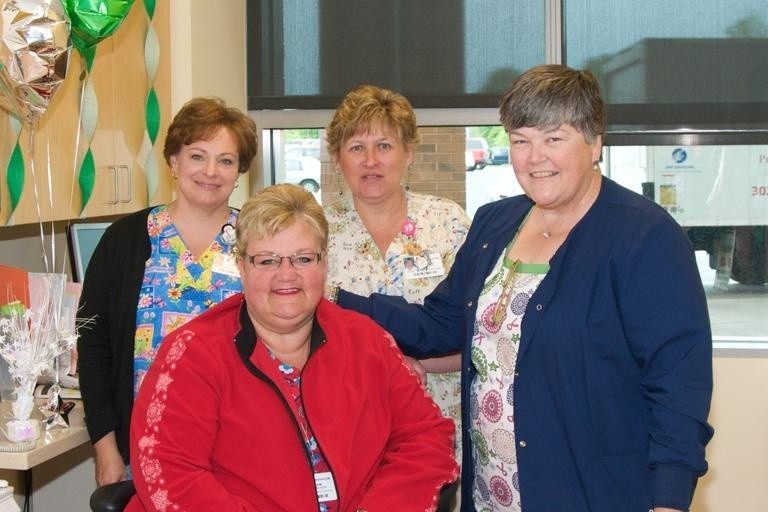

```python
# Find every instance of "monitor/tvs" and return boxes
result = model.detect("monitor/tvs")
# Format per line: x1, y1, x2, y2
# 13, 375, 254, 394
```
64, 221, 113, 283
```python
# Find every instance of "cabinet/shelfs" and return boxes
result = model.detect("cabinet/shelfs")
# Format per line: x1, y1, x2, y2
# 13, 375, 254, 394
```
0, 0, 174, 228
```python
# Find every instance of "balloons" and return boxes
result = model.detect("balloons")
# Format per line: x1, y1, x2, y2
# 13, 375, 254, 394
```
61, 0, 138, 59
1, 0, 74, 129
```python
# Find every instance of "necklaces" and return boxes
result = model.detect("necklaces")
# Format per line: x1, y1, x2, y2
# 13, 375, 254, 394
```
537, 190, 593, 240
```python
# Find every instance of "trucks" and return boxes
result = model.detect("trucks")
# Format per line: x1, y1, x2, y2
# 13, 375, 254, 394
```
599, 143, 767, 286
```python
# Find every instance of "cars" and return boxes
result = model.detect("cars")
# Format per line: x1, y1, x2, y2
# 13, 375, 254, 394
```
281, 139, 320, 195
462, 136, 508, 173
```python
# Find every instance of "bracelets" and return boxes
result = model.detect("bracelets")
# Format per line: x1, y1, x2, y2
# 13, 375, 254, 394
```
328, 287, 340, 304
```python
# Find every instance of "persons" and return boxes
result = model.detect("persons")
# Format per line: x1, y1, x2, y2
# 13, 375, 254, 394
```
321, 62, 715, 512
321, 83, 473, 511
405, 258, 415, 274
125, 182, 462, 511
74, 98, 260, 486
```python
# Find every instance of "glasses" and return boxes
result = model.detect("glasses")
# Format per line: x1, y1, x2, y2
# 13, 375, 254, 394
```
238, 251, 326, 269
492, 260, 524, 324
